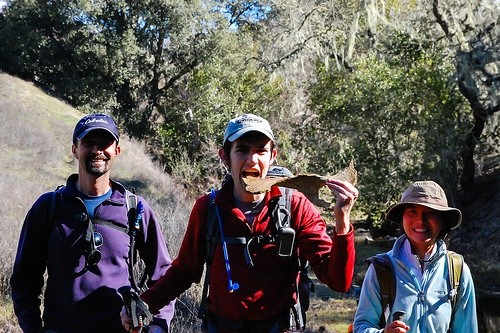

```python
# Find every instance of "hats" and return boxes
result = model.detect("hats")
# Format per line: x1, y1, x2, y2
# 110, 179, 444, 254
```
223, 113, 274, 147
385, 179, 463, 231
73, 113, 120, 142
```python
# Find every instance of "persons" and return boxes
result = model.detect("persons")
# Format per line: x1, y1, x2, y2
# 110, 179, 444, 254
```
353, 181, 478, 333
121, 113, 358, 333
10, 115, 176, 333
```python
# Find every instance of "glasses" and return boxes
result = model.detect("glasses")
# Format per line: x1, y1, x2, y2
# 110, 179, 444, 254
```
86, 220, 104, 268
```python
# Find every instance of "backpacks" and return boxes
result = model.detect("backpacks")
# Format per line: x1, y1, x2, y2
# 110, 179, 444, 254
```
204, 165, 315, 332
170, 283, 202, 333
353, 252, 464, 333
